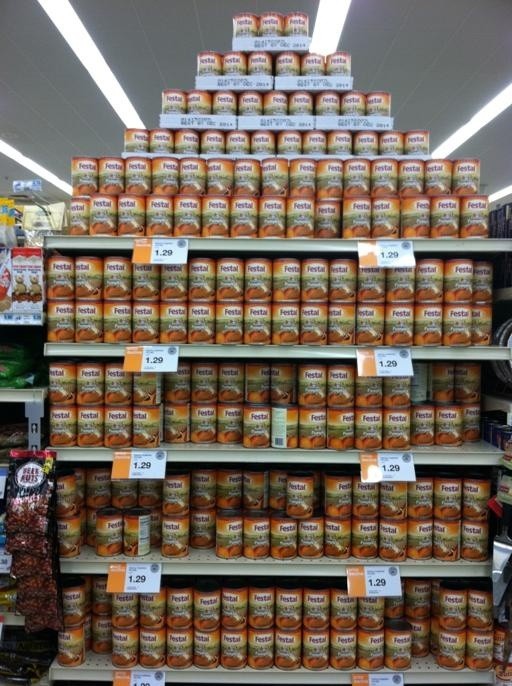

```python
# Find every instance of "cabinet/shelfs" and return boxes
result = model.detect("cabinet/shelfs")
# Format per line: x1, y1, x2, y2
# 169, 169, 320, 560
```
37, 237, 511, 686
0, 310, 48, 686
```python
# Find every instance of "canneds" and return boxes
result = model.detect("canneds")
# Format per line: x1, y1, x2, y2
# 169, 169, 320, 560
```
46, 255, 493, 346
196, 50, 351, 96
55, 468, 491, 562
232, 14, 309, 55
124, 126, 429, 155
67, 194, 489, 237
161, 88, 391, 136
58, 578, 509, 672
49, 363, 482, 452
71, 156, 480, 198
